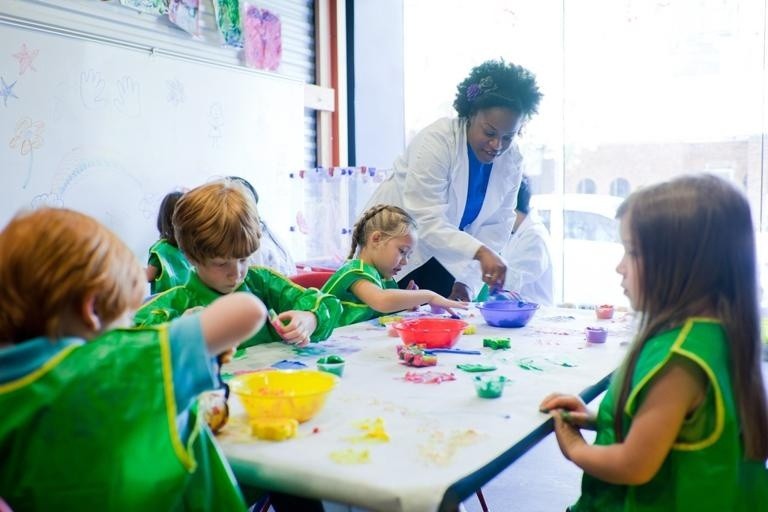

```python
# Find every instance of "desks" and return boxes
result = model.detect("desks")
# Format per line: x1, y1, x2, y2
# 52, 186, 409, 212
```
209, 303, 641, 512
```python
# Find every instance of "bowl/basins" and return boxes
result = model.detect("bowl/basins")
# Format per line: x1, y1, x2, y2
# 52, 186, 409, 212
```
315, 355, 347, 378
471, 375, 510, 399
474, 302, 541, 328
392, 317, 469, 350
585, 327, 609, 344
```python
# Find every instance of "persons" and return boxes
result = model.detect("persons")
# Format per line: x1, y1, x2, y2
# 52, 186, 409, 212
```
1, 209, 266, 512
226, 177, 297, 277
318, 204, 469, 328
502, 175, 560, 303
541, 176, 768, 511
132, 182, 343, 349
145, 192, 196, 295
360, 61, 541, 296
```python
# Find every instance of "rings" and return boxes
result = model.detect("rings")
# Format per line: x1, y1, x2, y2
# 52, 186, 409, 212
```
485, 273, 494, 280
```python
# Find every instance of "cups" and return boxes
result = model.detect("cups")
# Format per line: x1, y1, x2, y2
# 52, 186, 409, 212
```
229, 369, 342, 424
594, 306, 615, 321
